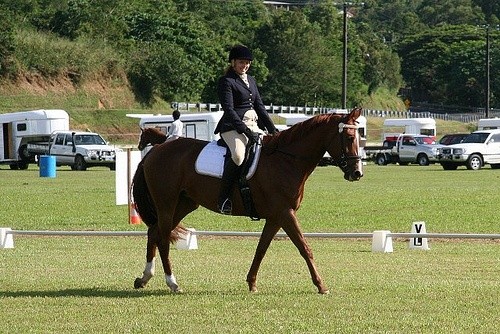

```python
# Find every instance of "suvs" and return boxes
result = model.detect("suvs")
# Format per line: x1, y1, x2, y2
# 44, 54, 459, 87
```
432, 129, 500, 171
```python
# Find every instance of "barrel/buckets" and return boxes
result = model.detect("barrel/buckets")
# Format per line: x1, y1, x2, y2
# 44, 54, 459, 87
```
39, 155, 56, 178
10, 161, 18, 170
17, 160, 28, 170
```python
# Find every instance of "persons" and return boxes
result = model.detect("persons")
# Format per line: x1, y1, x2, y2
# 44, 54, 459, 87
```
165, 110, 183, 142
214, 46, 279, 213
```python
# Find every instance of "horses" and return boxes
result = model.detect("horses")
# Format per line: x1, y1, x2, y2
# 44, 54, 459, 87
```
137, 125, 168, 151
129, 105, 364, 295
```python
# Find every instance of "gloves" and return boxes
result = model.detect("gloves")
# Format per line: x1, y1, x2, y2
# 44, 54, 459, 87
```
243, 127, 259, 145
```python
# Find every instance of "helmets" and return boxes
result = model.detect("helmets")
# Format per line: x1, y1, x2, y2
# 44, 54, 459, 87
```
229, 44, 253, 63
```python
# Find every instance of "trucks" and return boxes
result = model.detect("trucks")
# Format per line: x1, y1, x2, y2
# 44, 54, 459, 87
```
125, 109, 367, 166
0, 109, 69, 170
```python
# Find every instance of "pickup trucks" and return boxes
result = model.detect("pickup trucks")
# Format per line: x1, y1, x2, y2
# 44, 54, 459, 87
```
27, 129, 123, 171
364, 132, 439, 166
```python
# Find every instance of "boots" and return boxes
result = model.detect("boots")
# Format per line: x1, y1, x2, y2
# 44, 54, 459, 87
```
216, 158, 244, 213
